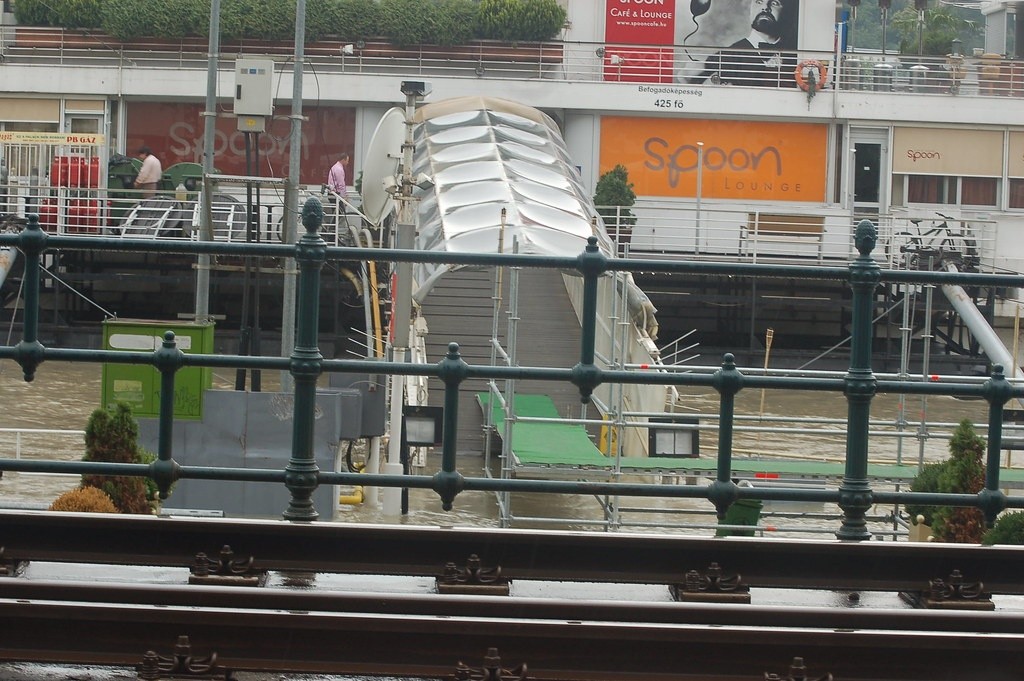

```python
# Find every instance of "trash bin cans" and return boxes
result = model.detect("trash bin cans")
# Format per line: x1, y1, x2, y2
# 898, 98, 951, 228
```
973, 48, 984, 55
910, 65, 930, 93
842, 59, 861, 90
873, 64, 894, 92
101, 317, 217, 421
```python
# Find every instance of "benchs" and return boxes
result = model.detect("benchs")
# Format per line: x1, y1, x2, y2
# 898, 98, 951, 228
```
738, 213, 827, 265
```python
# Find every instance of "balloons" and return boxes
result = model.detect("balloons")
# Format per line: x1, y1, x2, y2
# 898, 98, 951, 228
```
689, 0, 711, 15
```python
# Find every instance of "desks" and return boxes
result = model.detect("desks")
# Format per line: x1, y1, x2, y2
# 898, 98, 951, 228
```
108, 157, 203, 226
119, 196, 183, 239
182, 191, 247, 243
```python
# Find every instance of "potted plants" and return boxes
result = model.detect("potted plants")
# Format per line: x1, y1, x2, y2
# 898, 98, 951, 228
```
904, 458, 948, 542
889, 0, 990, 94
592, 163, 638, 253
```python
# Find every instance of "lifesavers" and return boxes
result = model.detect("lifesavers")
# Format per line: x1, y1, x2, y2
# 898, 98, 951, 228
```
795, 61, 828, 92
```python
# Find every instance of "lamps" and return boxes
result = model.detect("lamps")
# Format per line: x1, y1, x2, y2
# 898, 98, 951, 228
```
648, 417, 700, 458
403, 405, 443, 458
952, 38, 961, 56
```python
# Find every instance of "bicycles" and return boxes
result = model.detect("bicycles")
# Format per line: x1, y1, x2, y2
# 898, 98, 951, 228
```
883, 210, 970, 267
277, 202, 369, 247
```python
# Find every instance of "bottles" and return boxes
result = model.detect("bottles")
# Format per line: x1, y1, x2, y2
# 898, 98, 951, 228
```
176, 184, 187, 201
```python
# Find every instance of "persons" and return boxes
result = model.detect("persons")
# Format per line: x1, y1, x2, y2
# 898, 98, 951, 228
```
133, 146, 162, 199
326, 153, 348, 240
684, 0, 796, 88
